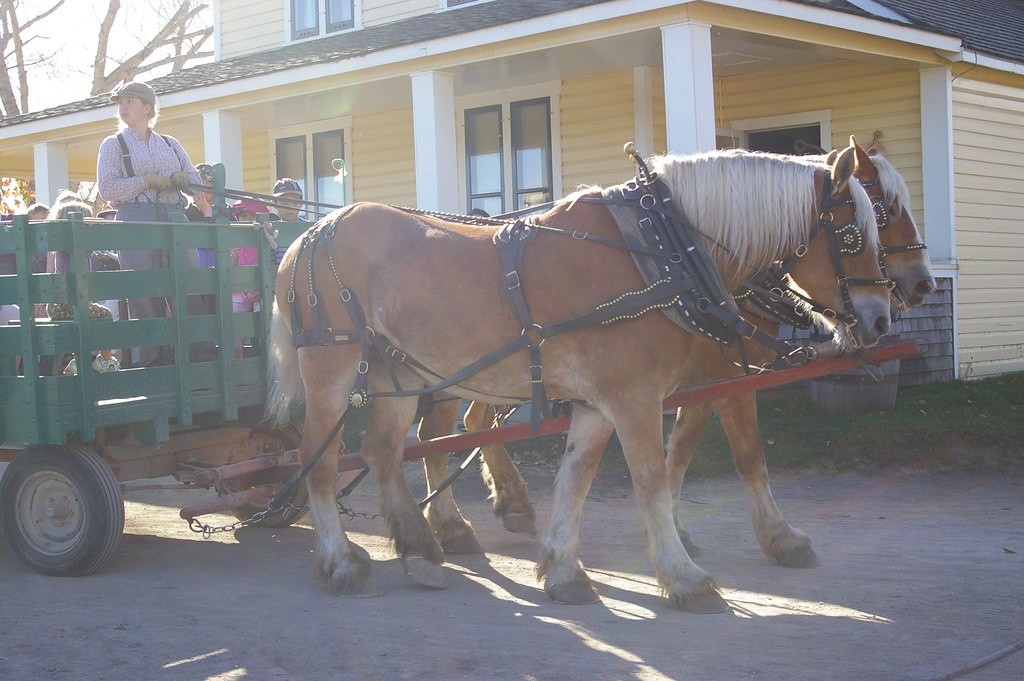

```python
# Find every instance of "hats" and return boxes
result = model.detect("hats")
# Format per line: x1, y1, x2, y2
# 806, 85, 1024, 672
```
232, 196, 270, 213
273, 178, 303, 198
195, 163, 213, 182
109, 82, 156, 105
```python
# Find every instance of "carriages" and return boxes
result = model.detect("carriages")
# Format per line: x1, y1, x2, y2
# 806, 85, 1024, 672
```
1, 132, 937, 613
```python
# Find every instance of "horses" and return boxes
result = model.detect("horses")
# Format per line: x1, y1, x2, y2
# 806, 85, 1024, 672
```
263, 135, 938, 616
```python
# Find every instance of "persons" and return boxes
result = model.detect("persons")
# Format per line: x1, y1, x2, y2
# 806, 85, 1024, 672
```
183, 163, 219, 315
0, 191, 278, 378
97, 81, 219, 363
269, 177, 311, 264
